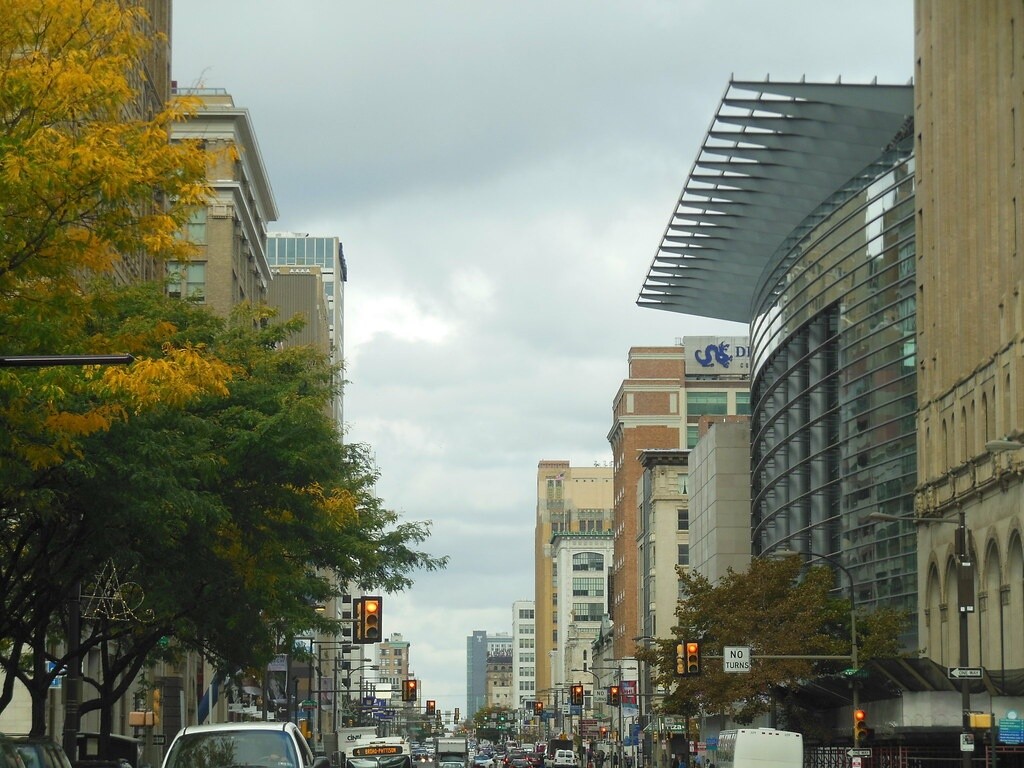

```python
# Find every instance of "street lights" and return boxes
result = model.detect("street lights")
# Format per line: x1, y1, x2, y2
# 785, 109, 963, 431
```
346, 664, 380, 703
869, 509, 976, 767
554, 681, 584, 768
587, 667, 637, 768
318, 657, 372, 740
600, 657, 646, 768
632, 634, 691, 768
774, 547, 861, 749
307, 639, 354, 747
317, 646, 360, 743
570, 669, 602, 742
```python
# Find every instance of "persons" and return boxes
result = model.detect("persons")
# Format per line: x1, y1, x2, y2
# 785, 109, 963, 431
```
576, 745, 711, 768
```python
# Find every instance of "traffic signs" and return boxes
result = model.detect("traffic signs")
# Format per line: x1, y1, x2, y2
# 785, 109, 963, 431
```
947, 667, 984, 680
844, 748, 873, 759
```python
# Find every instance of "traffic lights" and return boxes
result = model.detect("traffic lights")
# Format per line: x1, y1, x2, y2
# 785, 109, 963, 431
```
537, 701, 543, 715
500, 712, 505, 721
574, 685, 585, 705
407, 680, 416, 702
854, 710, 866, 741
683, 639, 702, 677
348, 719, 353, 729
609, 686, 619, 707
361, 596, 385, 644
484, 712, 488, 721
601, 727, 607, 740
429, 700, 435, 715
498, 722, 502, 731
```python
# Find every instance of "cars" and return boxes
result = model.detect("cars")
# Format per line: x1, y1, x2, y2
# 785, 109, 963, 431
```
0, 721, 578, 768
